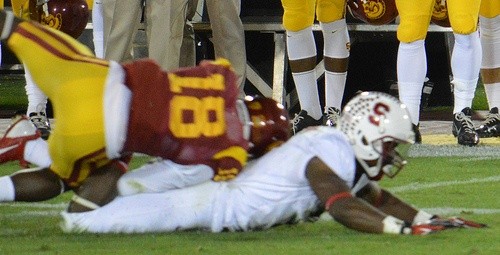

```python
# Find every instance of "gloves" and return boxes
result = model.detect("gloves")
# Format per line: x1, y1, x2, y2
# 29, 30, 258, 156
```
383, 214, 443, 235
412, 209, 486, 229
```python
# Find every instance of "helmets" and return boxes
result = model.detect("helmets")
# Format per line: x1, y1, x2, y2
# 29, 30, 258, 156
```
38, 0, 89, 40
431, 0, 452, 27
335, 91, 414, 181
246, 97, 288, 154
347, 0, 398, 25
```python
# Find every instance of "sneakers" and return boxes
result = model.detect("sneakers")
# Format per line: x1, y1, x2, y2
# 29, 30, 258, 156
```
29, 111, 51, 139
452, 107, 479, 147
324, 107, 341, 126
411, 122, 422, 144
475, 107, 500, 138
288, 109, 323, 136
0, 114, 41, 168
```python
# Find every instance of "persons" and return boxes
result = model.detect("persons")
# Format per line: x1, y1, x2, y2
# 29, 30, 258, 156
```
0, 0, 245, 140
60, 92, 486, 235
281, 0, 350, 135
476, 0, 500, 137
0, 7, 290, 201
396, 0, 481, 146
